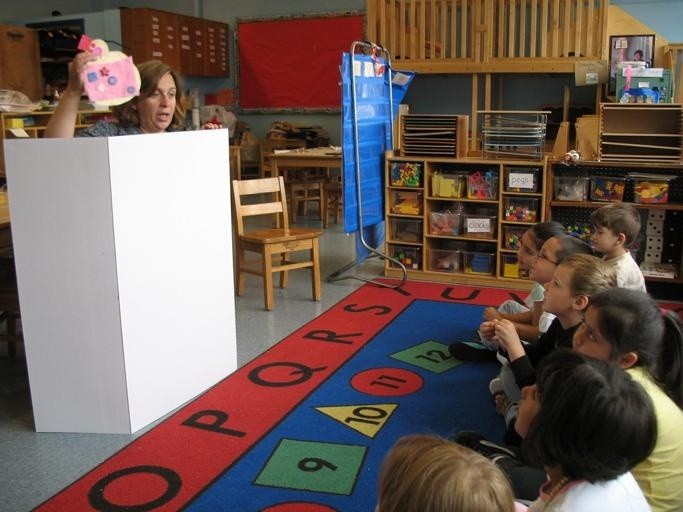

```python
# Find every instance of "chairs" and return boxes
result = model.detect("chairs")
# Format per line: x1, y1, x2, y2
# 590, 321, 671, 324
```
322, 172, 343, 229
258, 141, 324, 227
250, 142, 275, 186
229, 174, 325, 310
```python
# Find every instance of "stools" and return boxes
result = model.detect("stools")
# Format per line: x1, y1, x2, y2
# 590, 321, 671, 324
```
0, 248, 24, 364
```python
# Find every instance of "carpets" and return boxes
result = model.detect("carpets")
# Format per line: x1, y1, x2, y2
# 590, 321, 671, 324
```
63, 279, 683, 512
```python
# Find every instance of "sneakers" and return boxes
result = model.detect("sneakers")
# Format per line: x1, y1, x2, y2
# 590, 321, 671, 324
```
449, 338, 493, 363
460, 432, 506, 463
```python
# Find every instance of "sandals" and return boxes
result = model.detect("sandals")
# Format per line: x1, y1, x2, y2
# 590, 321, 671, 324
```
492, 389, 516, 415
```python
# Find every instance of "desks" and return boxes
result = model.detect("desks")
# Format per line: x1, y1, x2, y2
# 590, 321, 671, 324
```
268, 145, 343, 230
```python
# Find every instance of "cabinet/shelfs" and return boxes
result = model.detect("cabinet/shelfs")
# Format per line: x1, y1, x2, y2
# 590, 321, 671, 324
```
375, 120, 568, 289
121, 7, 180, 73
0, 103, 116, 155
552, 102, 683, 292
176, 14, 207, 77
207, 21, 232, 78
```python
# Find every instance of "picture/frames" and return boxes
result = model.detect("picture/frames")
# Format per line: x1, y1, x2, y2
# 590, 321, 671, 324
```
607, 35, 657, 97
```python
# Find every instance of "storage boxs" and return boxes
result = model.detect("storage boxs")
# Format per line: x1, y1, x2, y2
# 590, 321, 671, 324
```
500, 255, 522, 278
390, 218, 424, 245
428, 209, 463, 239
503, 226, 528, 250
610, 65, 670, 103
462, 213, 500, 239
390, 164, 420, 187
503, 167, 540, 193
464, 171, 500, 201
431, 172, 465, 200
463, 246, 496, 277
390, 191, 424, 215
555, 173, 589, 202
387, 245, 421, 271
504, 196, 538, 223
589, 172, 630, 203
429, 246, 463, 272
627, 170, 676, 204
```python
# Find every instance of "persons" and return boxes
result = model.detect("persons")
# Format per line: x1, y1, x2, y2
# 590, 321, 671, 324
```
509, 347, 658, 511
589, 200, 647, 291
43, 51, 222, 139
455, 289, 683, 512
374, 434, 515, 512
634, 50, 642, 61
488, 232, 593, 397
479, 220, 566, 350
479, 254, 618, 429
